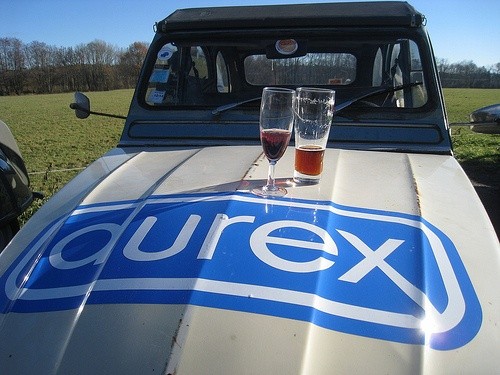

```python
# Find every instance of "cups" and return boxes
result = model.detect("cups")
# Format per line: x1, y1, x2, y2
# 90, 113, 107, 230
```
292, 87, 335, 185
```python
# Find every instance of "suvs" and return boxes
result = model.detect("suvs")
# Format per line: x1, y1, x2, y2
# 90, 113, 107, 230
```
0, 2, 500, 375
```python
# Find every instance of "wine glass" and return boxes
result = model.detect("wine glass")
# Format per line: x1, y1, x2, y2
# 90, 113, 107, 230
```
250, 87, 295, 198
